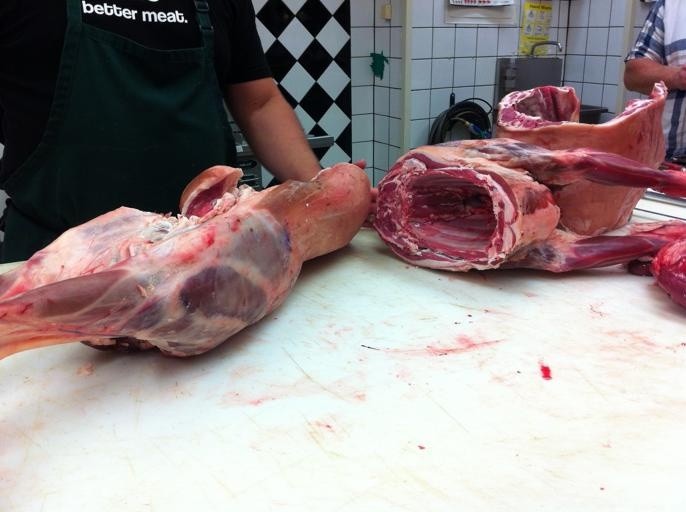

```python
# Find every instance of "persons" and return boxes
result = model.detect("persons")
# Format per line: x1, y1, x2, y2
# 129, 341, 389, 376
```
624, 0, 686, 170
0, 0, 379, 264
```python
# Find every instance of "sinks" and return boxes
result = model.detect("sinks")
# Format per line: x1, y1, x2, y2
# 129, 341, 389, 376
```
579, 104, 609, 124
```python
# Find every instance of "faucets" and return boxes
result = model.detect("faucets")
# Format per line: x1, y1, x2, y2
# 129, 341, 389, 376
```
531, 41, 562, 58
